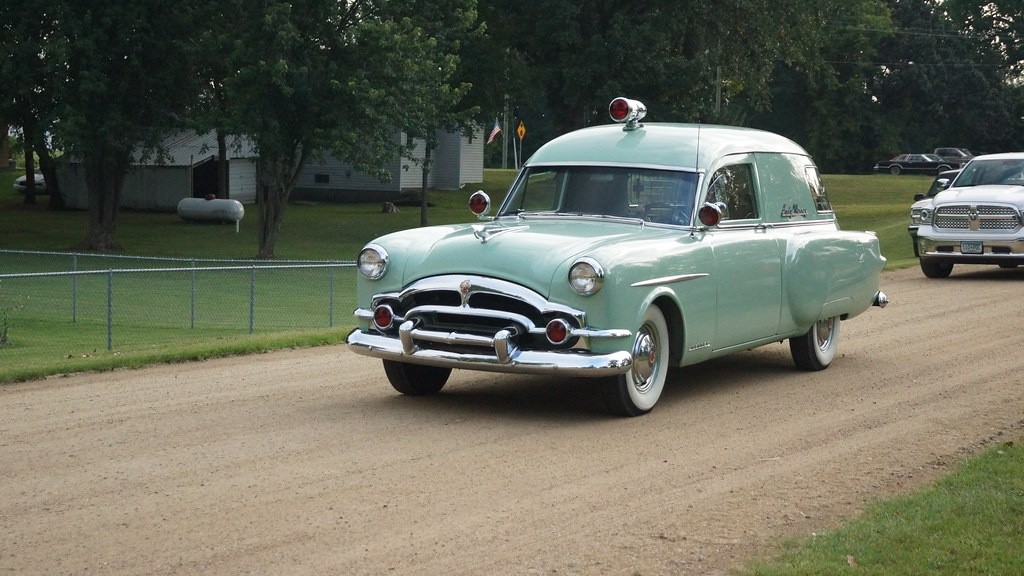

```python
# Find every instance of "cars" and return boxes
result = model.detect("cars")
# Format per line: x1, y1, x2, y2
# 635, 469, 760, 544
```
344, 94, 890, 418
13, 167, 49, 194
933, 148, 976, 169
874, 153, 967, 175
910, 169, 977, 257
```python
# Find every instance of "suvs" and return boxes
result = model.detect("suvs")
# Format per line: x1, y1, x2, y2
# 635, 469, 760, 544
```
916, 152, 1024, 279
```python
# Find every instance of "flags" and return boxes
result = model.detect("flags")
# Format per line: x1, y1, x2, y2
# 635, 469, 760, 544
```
487, 117, 500, 145
